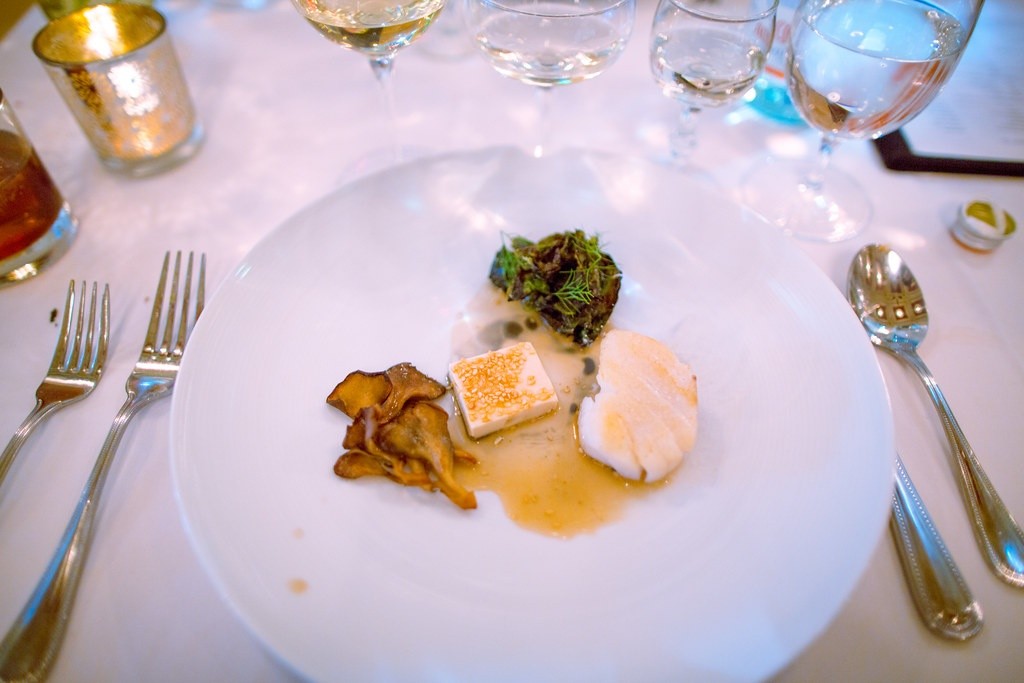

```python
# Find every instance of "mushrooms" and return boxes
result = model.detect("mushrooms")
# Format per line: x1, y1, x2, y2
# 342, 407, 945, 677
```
324, 361, 478, 510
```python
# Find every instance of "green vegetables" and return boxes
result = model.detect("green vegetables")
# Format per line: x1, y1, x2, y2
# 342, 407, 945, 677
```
495, 226, 621, 320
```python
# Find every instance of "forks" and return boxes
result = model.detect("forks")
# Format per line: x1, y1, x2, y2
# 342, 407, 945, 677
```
0, 280, 111, 483
0, 251, 205, 682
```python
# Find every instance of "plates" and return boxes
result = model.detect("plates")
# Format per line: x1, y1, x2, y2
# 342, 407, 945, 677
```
169, 144, 896, 683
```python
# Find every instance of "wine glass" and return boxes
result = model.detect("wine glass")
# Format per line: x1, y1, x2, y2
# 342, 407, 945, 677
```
650, 0, 779, 157
733, 0, 987, 239
294, 1, 444, 123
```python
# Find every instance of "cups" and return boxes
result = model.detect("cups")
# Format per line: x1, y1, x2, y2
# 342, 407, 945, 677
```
464, 0, 635, 86
0, 89, 77, 286
32, 3, 202, 177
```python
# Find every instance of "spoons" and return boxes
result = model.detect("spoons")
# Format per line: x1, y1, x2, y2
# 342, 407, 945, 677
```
848, 244, 1024, 589
